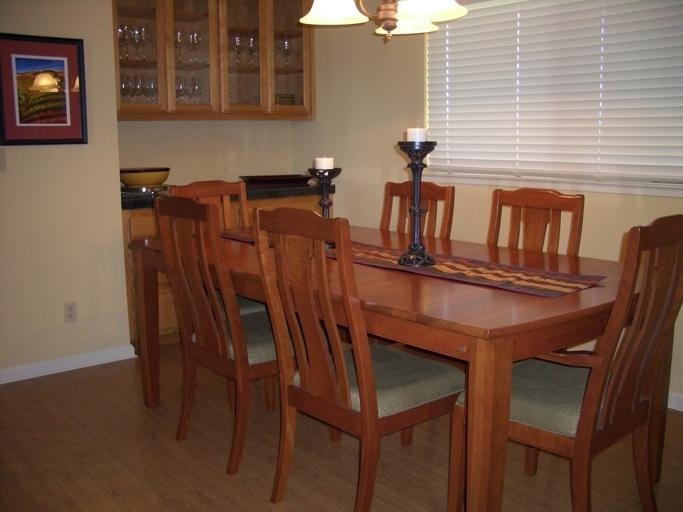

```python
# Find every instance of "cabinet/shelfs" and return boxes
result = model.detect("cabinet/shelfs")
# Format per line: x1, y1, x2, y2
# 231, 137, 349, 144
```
122, 193, 334, 345
113, 1, 316, 121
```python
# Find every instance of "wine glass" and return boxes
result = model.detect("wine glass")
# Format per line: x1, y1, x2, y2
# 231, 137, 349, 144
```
176, 76, 202, 104
121, 73, 158, 104
228, 34, 257, 67
279, 36, 293, 68
118, 25, 156, 61
175, 29, 202, 64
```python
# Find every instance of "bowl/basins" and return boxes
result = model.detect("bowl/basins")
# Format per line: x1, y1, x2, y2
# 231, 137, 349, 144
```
120, 167, 170, 189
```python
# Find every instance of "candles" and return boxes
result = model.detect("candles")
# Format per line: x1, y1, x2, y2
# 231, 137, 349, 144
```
314, 154, 334, 169
407, 126, 428, 141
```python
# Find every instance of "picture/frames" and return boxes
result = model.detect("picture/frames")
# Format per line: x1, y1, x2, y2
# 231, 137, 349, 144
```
0, 33, 88, 145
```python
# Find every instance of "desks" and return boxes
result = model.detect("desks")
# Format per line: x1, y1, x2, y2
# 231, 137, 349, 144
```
128, 218, 668, 512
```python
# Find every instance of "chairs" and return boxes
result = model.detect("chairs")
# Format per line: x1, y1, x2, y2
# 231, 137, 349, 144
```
165, 178, 251, 231
252, 204, 466, 511
153, 197, 280, 475
379, 181, 454, 243
488, 187, 583, 255
448, 215, 681, 510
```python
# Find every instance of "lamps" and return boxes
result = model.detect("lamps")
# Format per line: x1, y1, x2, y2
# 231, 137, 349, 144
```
298, 1, 468, 41
